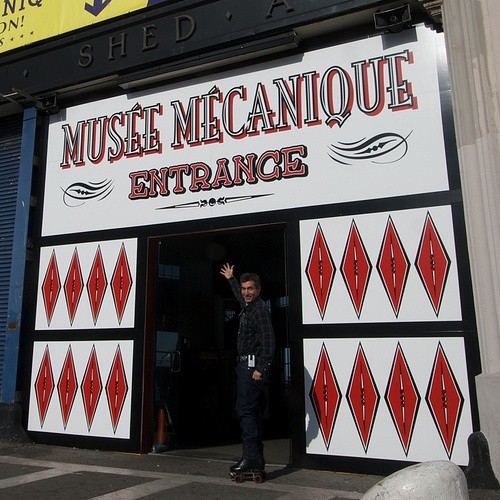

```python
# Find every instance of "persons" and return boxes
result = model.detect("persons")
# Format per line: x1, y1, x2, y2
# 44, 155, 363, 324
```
220, 262, 273, 473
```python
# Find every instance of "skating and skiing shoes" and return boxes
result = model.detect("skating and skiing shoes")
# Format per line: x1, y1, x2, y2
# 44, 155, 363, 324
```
229, 457, 265, 483
